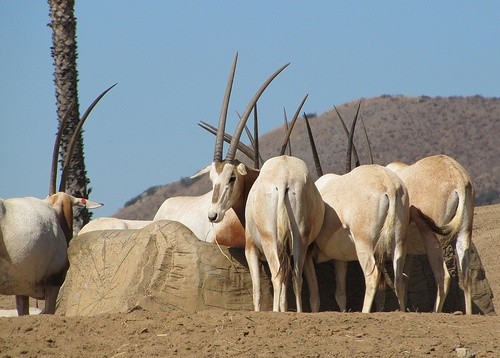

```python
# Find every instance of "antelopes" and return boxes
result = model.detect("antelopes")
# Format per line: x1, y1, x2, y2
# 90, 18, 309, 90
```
77, 50, 476, 314
0, 82, 118, 316
